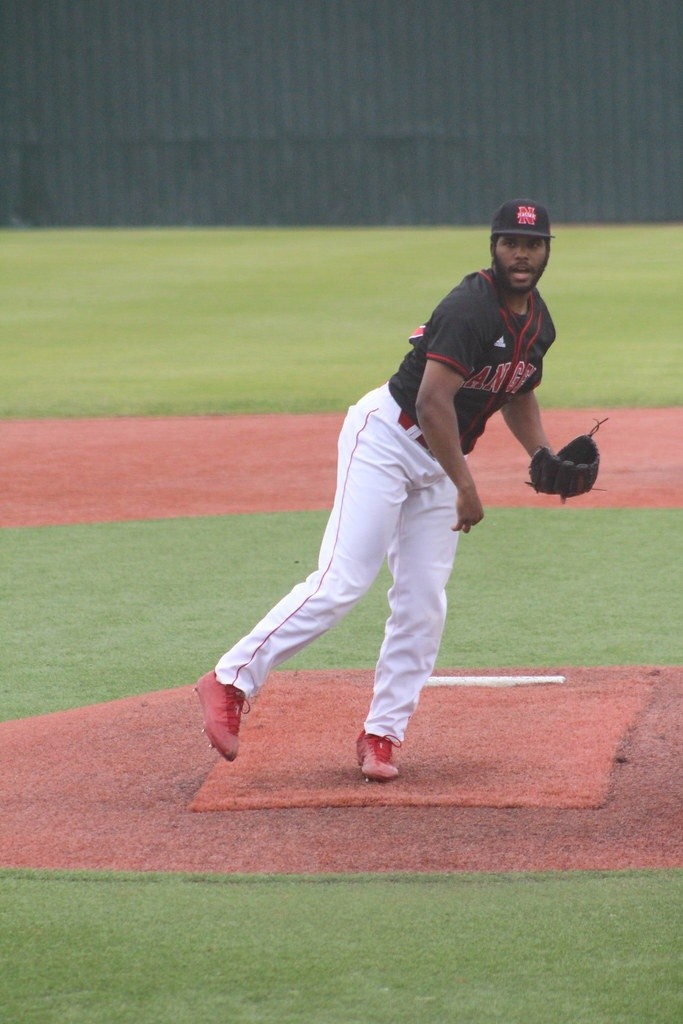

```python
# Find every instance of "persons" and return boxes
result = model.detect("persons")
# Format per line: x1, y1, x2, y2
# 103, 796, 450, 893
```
194, 199, 555, 782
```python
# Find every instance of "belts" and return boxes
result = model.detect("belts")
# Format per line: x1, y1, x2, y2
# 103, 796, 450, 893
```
399, 409, 430, 449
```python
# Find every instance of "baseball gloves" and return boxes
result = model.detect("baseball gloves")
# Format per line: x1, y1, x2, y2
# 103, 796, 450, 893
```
527, 434, 599, 497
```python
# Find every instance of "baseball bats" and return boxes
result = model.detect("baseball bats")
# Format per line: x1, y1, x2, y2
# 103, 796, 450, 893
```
424, 674, 566, 686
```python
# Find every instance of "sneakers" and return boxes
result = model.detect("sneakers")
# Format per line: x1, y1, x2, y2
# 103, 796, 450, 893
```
356, 730, 402, 783
193, 671, 251, 760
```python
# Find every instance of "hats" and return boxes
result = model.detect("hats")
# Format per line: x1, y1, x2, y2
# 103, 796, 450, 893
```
492, 199, 555, 238
